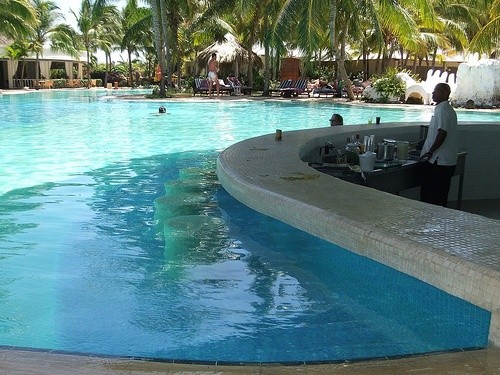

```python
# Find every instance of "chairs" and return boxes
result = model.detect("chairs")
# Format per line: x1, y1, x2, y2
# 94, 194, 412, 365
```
227, 77, 252, 96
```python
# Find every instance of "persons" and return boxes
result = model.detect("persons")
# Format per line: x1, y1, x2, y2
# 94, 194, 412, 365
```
421, 83, 458, 205
329, 114, 343, 126
159, 106, 166, 113
206, 53, 223, 99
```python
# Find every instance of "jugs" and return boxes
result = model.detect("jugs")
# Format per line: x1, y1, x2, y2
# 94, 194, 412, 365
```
397, 140, 409, 160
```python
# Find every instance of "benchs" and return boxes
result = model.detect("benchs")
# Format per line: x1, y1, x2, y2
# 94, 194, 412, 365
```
313, 84, 336, 97
193, 77, 234, 97
268, 80, 311, 98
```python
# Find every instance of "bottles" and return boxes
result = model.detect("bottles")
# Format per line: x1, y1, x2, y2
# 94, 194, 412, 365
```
354, 135, 362, 153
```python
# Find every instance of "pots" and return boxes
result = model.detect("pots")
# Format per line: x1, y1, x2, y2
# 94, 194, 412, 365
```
377, 142, 397, 161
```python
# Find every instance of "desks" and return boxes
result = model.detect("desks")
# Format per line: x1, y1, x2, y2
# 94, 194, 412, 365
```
361, 149, 468, 201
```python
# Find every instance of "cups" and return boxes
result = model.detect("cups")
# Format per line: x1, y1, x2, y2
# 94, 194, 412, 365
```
275, 129, 282, 141
367, 116, 372, 125
363, 135, 376, 152
376, 116, 380, 124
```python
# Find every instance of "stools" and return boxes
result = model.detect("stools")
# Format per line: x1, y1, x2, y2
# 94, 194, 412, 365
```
234, 86, 241, 95
